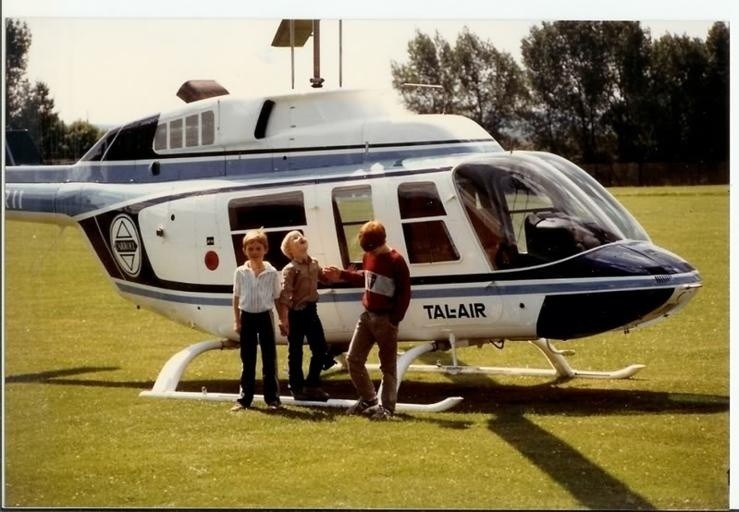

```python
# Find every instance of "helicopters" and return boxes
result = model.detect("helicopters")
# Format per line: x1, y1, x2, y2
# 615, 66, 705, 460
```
3, 18, 702, 427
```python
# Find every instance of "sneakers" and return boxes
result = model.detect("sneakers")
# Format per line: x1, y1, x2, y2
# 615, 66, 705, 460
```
345, 397, 394, 421
230, 402, 243, 411
288, 384, 332, 402
267, 404, 277, 410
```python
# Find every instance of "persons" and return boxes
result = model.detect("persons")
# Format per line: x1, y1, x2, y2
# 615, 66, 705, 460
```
230, 225, 289, 411
278, 230, 357, 400
323, 220, 411, 418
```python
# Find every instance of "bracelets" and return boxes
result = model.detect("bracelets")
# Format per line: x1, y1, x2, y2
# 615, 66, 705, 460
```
234, 320, 240, 322
